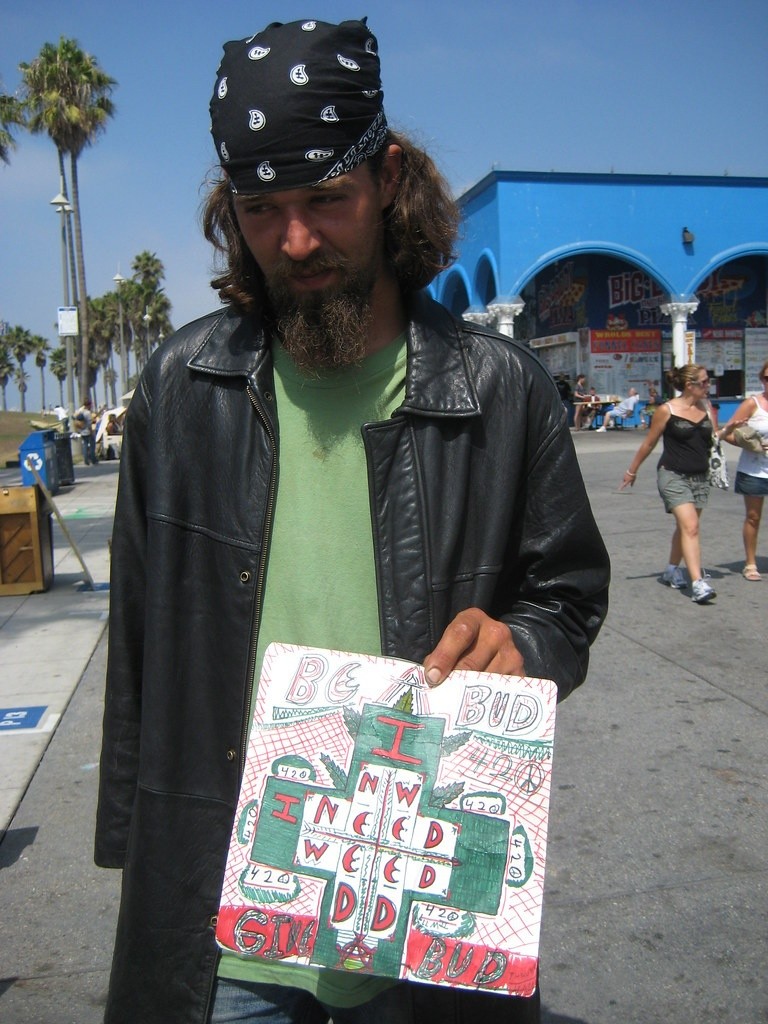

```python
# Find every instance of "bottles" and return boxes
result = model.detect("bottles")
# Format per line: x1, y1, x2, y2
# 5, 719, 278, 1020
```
591, 395, 595, 402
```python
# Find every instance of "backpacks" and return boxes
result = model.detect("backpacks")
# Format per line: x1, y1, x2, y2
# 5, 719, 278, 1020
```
72, 407, 87, 433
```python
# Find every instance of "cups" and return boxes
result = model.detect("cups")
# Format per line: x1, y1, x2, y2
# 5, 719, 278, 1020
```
606, 396, 610, 402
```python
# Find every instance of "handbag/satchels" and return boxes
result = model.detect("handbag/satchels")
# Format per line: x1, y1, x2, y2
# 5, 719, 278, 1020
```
708, 432, 731, 489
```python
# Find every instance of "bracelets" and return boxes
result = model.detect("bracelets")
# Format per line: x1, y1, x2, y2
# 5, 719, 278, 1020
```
722, 428, 731, 435
626, 470, 636, 476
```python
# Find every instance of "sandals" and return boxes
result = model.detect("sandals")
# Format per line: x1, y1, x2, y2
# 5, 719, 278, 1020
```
742, 565, 761, 580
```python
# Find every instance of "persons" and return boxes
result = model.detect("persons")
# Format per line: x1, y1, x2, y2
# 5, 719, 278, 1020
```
619, 365, 748, 602
723, 362, 768, 581
78, 398, 99, 466
107, 414, 123, 435
41, 406, 46, 421
556, 373, 574, 427
54, 406, 69, 433
92, 20, 614, 1024
649, 388, 663, 428
596, 388, 636, 432
574, 374, 600, 431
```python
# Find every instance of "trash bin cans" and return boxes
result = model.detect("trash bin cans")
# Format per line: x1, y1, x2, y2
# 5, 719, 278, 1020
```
18, 427, 74, 497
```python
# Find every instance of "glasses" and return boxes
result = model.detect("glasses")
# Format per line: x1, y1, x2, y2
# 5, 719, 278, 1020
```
763, 376, 768, 381
691, 378, 710, 387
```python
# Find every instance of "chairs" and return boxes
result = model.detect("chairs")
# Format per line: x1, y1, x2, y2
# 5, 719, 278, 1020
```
580, 403, 638, 430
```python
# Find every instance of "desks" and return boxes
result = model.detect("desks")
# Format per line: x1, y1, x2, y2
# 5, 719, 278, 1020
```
574, 401, 619, 427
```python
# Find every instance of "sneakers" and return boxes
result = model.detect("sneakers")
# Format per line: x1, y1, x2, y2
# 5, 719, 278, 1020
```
662, 564, 689, 588
692, 569, 718, 602
595, 426, 607, 432
609, 420, 614, 428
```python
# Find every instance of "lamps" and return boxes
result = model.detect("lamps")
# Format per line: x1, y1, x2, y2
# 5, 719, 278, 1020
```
682, 227, 694, 245
